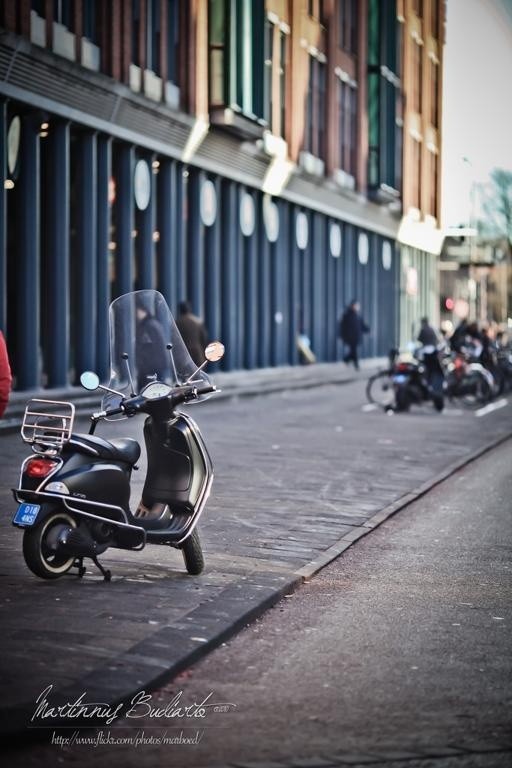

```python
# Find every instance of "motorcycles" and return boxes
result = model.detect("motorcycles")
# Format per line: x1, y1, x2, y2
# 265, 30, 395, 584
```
9, 288, 227, 583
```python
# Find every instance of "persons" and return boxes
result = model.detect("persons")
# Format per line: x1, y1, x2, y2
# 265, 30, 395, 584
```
133, 302, 167, 394
417, 318, 438, 351
339, 300, 372, 372
173, 297, 206, 399
1, 330, 14, 419
